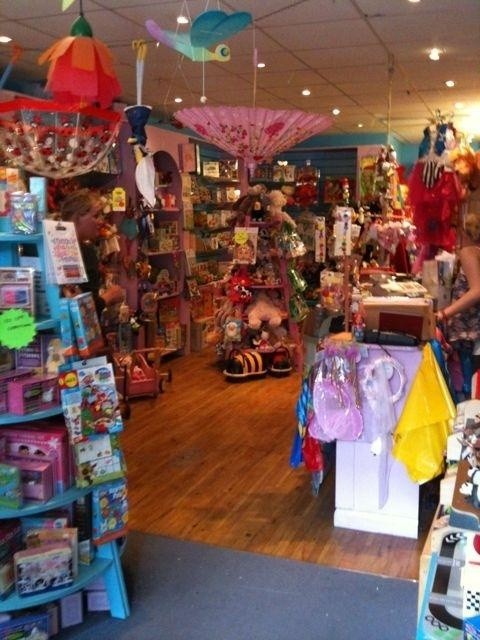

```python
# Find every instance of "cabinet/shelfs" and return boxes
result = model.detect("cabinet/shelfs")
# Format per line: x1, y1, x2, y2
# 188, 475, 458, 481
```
111, 122, 194, 358
0, 171, 131, 622
224, 215, 301, 372
328, 276, 434, 539
238, 146, 390, 231
177, 135, 248, 355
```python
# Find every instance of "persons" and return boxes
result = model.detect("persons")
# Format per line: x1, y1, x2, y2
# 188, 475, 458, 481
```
61, 190, 104, 322
435, 212, 480, 400
123, 38, 156, 209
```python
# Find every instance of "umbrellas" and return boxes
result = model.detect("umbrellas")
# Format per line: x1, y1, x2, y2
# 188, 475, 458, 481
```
172, 49, 337, 178
0, 100, 122, 182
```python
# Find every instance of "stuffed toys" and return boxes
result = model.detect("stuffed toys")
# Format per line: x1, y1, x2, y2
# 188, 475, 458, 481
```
99, 187, 181, 353
224, 184, 268, 228
265, 190, 297, 229
348, 145, 417, 341
202, 264, 288, 353
457, 410, 480, 509
